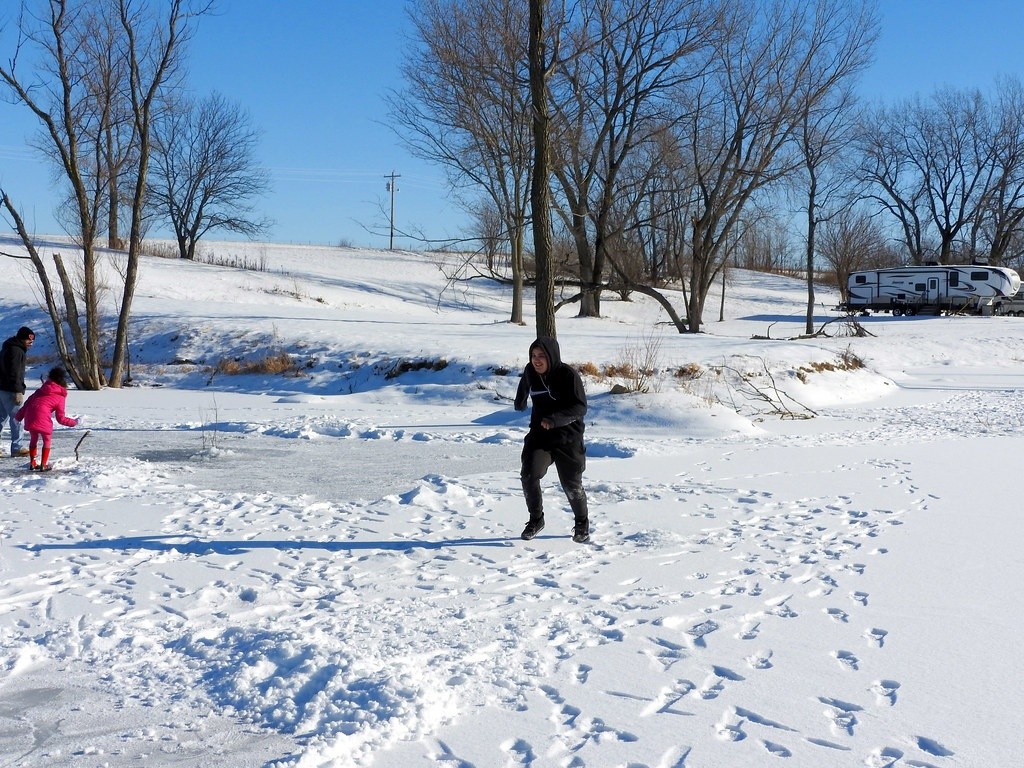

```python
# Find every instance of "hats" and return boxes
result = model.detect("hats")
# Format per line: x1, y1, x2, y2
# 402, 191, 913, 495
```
17, 327, 35, 341
47, 366, 68, 378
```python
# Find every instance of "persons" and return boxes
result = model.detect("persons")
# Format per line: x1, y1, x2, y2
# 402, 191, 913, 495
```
0, 326, 36, 458
512, 336, 592, 544
15, 365, 82, 472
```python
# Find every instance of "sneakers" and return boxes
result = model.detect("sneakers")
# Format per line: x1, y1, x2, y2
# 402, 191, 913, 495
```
12, 449, 29, 457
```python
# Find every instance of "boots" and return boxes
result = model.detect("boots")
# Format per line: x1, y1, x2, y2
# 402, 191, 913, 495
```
30, 446, 41, 471
572, 519, 590, 543
522, 513, 545, 540
41, 447, 51, 472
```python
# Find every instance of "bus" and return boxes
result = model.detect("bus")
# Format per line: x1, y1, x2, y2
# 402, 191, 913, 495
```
842, 264, 1019, 316
995, 281, 1024, 317
